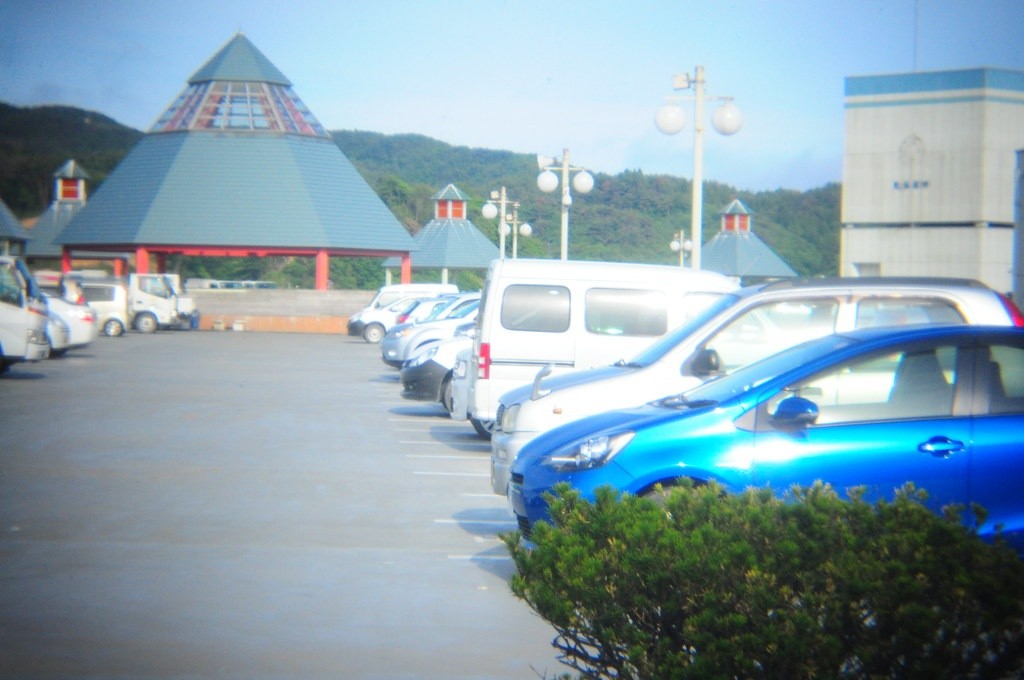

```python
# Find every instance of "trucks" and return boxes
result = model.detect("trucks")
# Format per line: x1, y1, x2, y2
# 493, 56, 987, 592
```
58, 271, 201, 337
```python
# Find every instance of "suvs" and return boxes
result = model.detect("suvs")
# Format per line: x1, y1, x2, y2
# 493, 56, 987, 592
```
0, 251, 52, 376
488, 275, 1024, 503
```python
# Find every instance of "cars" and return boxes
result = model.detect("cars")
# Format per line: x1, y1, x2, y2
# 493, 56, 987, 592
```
45, 310, 69, 355
381, 293, 621, 419
42, 288, 98, 350
508, 317, 1024, 571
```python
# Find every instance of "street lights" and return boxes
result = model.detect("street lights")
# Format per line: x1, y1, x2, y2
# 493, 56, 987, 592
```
536, 147, 595, 261
670, 229, 692, 267
482, 186, 518, 258
656, 65, 744, 271
497, 209, 533, 258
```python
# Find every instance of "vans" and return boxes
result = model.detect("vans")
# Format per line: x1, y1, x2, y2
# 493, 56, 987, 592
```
348, 283, 460, 345
466, 258, 869, 446
